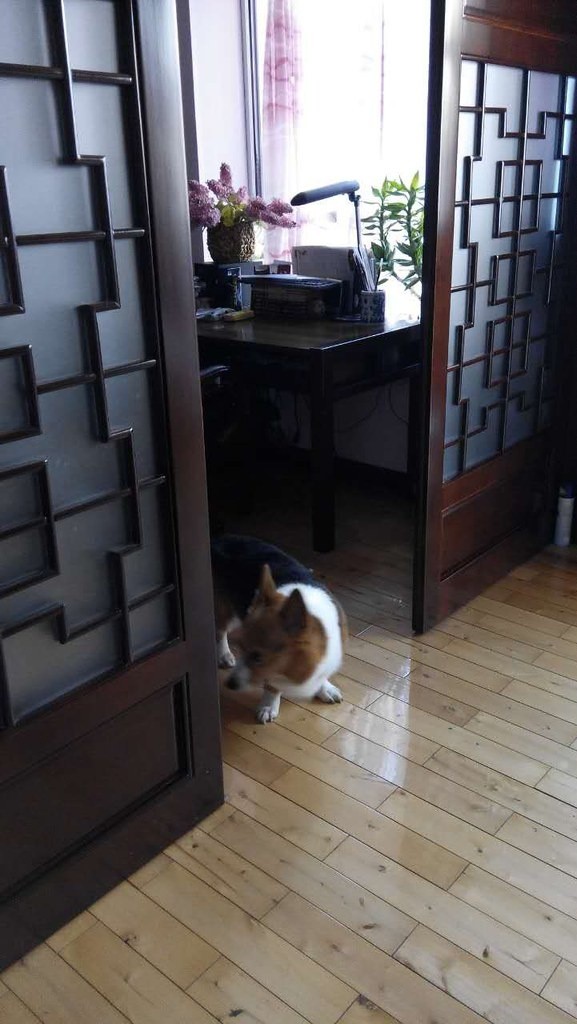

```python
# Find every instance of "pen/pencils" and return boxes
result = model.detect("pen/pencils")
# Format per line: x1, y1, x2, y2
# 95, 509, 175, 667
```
352, 244, 383, 291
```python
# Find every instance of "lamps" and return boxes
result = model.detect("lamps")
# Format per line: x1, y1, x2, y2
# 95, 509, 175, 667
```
292, 175, 371, 322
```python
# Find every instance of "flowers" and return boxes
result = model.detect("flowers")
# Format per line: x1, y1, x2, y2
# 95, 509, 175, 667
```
189, 162, 304, 230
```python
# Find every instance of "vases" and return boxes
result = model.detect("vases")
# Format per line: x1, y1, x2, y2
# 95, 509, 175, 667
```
205, 219, 256, 264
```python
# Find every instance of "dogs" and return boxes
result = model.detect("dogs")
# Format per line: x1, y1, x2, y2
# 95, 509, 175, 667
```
211, 529, 349, 725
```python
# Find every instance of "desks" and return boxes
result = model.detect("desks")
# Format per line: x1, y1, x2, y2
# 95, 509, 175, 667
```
197, 310, 422, 552
